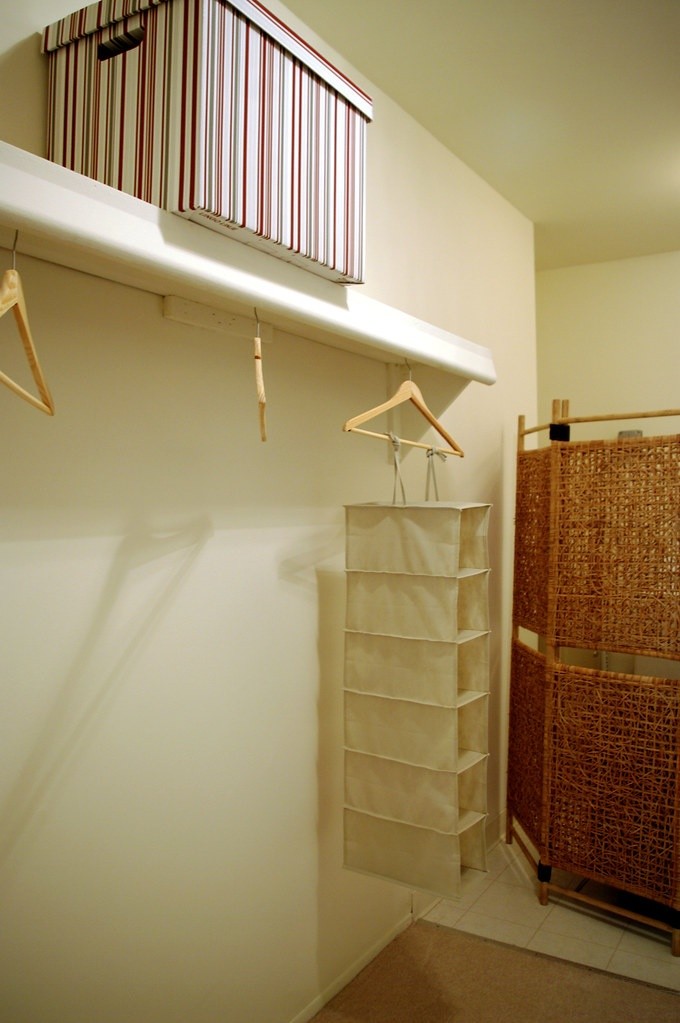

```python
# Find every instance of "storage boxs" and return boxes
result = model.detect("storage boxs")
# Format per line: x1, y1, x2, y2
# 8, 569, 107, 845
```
40, 0, 376, 286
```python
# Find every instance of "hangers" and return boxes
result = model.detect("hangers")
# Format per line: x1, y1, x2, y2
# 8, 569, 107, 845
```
253, 306, 270, 443
0, 229, 54, 415
341, 355, 465, 459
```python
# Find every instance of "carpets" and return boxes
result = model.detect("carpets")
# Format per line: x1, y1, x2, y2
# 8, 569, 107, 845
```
305, 919, 679, 1023
